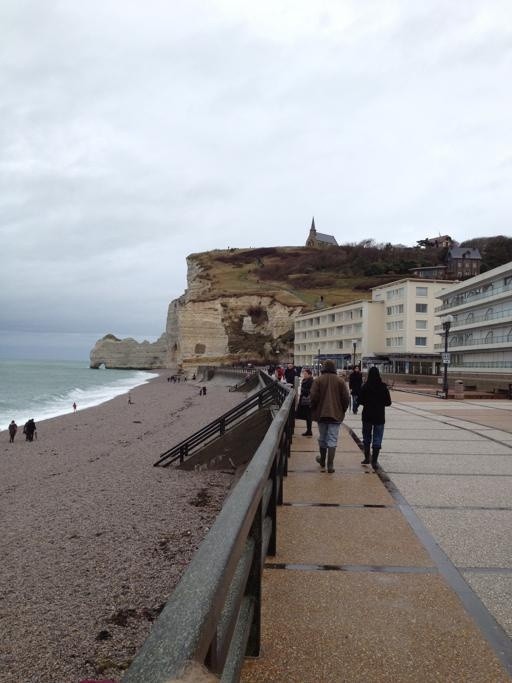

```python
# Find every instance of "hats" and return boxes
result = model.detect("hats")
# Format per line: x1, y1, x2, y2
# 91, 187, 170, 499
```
321, 359, 337, 372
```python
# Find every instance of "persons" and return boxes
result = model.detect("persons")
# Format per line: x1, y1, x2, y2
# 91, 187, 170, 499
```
73, 402, 76, 413
309, 358, 351, 472
349, 365, 363, 414
23, 419, 32, 440
266, 360, 303, 387
165, 373, 196, 383
28, 418, 36, 441
296, 368, 314, 435
355, 366, 392, 468
8, 420, 17, 442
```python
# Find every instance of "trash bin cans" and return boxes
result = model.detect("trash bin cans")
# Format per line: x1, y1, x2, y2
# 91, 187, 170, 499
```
454, 379, 465, 400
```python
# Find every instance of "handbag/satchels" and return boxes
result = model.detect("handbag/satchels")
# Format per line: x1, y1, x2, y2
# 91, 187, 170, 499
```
301, 396, 312, 406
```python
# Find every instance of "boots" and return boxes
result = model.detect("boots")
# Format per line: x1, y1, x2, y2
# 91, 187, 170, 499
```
372, 447, 379, 468
303, 416, 312, 436
328, 447, 335, 473
316, 446, 326, 466
361, 445, 370, 464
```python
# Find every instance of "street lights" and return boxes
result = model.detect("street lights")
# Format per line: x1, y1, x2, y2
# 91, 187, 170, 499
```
439, 315, 454, 397
352, 339, 360, 374
275, 349, 281, 365
